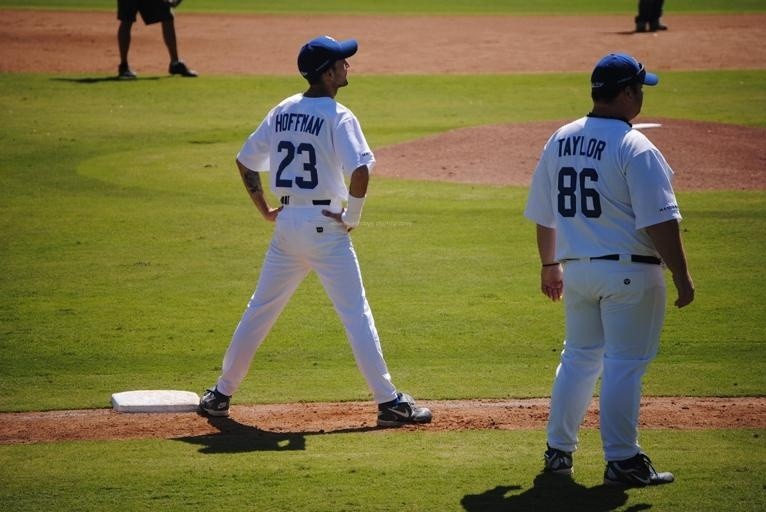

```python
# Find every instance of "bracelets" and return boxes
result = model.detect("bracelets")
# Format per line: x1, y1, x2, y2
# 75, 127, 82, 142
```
538, 261, 561, 269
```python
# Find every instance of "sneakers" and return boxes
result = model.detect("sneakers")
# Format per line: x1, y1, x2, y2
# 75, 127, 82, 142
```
377, 393, 432, 428
119, 65, 135, 77
635, 13, 647, 33
169, 63, 196, 76
604, 452, 674, 487
544, 443, 574, 478
200, 385, 232, 417
648, 18, 669, 31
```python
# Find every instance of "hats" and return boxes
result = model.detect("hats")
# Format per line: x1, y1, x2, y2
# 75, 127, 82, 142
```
591, 52, 658, 90
297, 35, 358, 80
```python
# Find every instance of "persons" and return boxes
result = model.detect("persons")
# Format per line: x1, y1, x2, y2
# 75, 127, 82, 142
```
115, 0, 200, 81
633, 0, 668, 32
196, 32, 433, 428
524, 50, 695, 491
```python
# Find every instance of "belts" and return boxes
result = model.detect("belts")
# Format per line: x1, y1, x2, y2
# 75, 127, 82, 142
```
280, 196, 331, 206
564, 254, 661, 266
